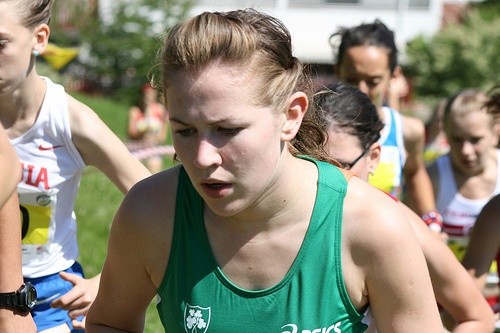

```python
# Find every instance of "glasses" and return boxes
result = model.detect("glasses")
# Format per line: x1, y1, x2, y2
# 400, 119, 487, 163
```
342, 151, 366, 170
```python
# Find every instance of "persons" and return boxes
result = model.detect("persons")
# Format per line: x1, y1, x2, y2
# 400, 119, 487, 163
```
0, 121, 38, 333
84, 10, 500, 333
0, 0, 154, 333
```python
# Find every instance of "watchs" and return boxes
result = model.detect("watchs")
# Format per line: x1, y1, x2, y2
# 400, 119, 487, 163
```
0, 282, 38, 313
